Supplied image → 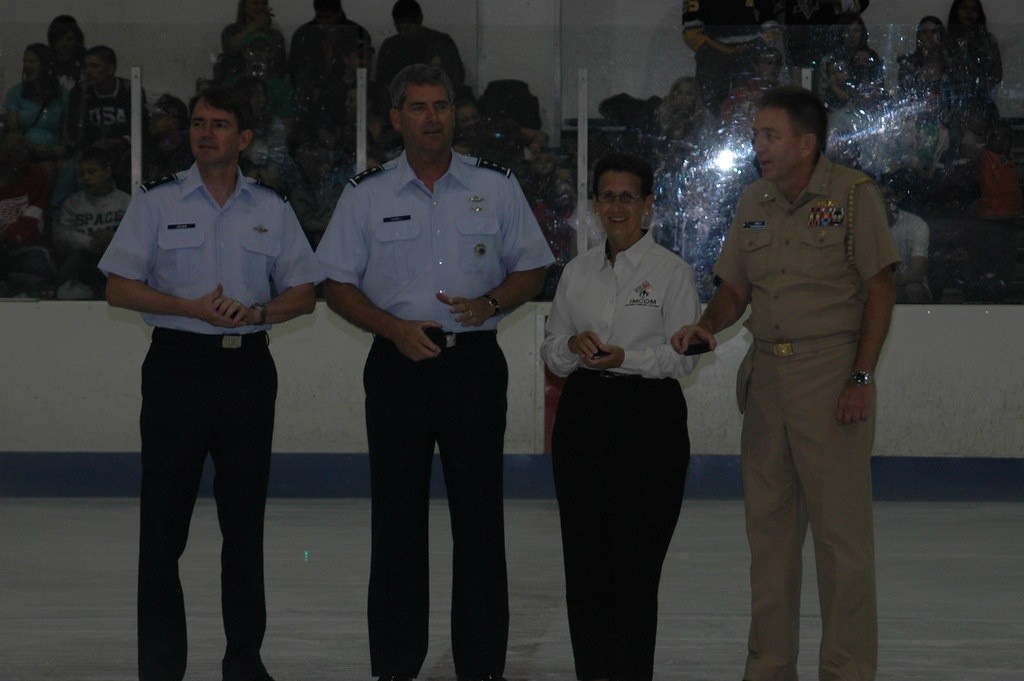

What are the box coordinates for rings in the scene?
[469,309,473,316]
[234,301,240,306]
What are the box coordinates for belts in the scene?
[754,330,857,359]
[577,366,641,379]
[374,327,498,348]
[151,325,266,349]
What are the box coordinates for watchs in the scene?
[850,371,872,386]
[483,294,499,316]
[252,302,265,325]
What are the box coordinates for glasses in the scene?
[598,190,641,204]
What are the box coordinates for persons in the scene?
[98,88,326,681]
[1,0,1024,305]
[310,62,555,681]
[670,86,903,681]
[540,154,703,681]
